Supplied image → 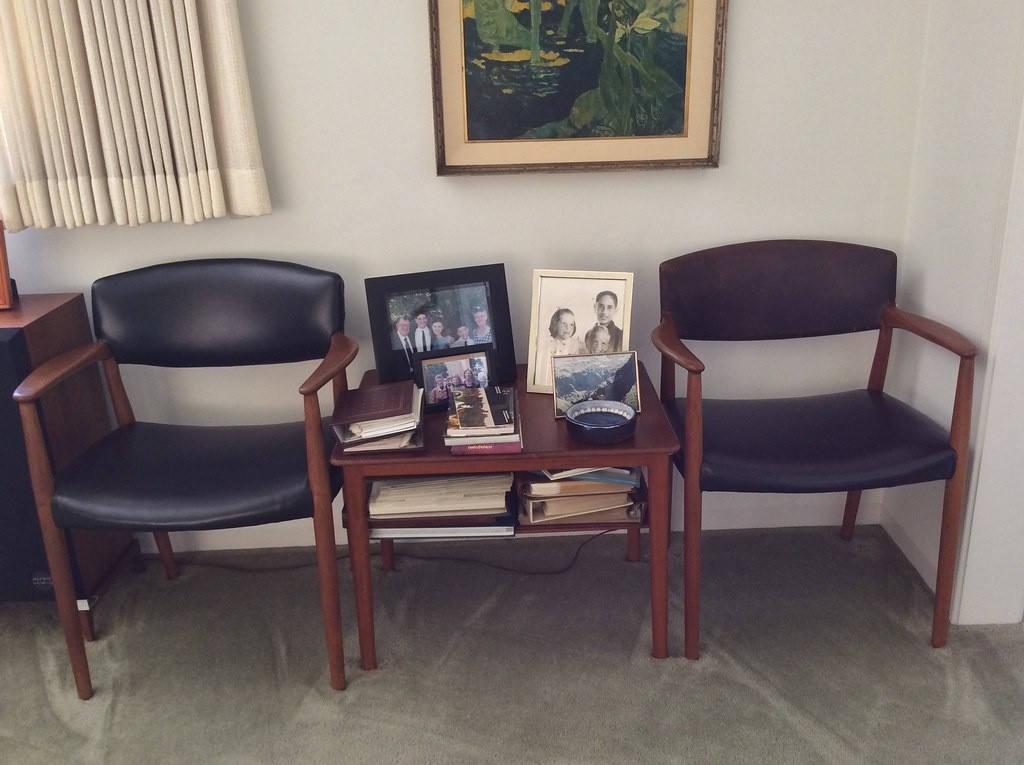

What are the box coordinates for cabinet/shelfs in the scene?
[0,292,147,644]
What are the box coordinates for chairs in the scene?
[14,256,359,701]
[649,239,978,659]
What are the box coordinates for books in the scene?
[443,387,524,454]
[329,382,424,453]
[366,467,643,538]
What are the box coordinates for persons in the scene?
[584,291,622,354]
[431,370,489,404]
[391,305,493,380]
[535,309,578,387]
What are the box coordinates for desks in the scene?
[328,361,682,667]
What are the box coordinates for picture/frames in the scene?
[364,262,518,386]
[526,269,635,394]
[552,350,642,419]
[428,0,730,178]
[410,343,498,414]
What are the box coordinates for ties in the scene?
[404,338,414,366]
[465,341,467,346]
[422,330,427,351]
